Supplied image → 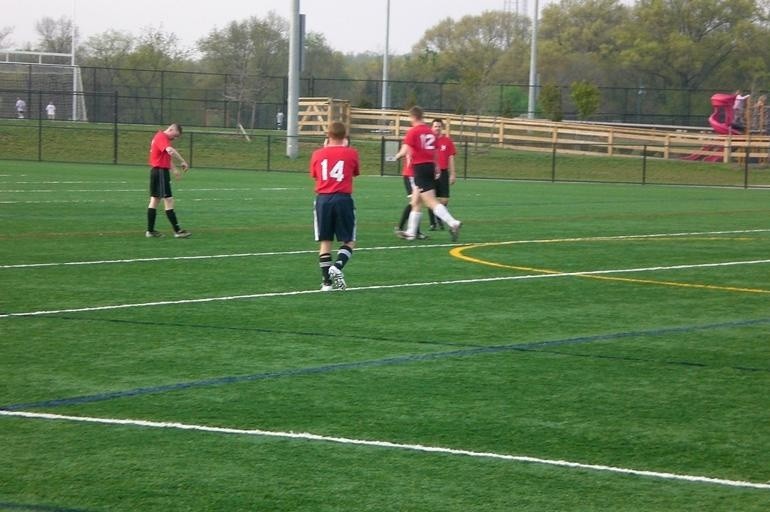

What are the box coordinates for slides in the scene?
[678,94,744,161]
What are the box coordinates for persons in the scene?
[385,137,431,239]
[311,123,360,291]
[276,109,285,130]
[145,123,192,237]
[397,106,462,241]
[45,102,56,119]
[427,119,456,231]
[15,97,25,118]
[733,89,750,122]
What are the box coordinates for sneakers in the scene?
[328,265,347,291]
[396,231,417,240]
[449,220,462,241]
[429,218,444,231]
[417,232,426,239]
[146,230,164,237]
[320,283,332,290]
[174,229,192,238]
